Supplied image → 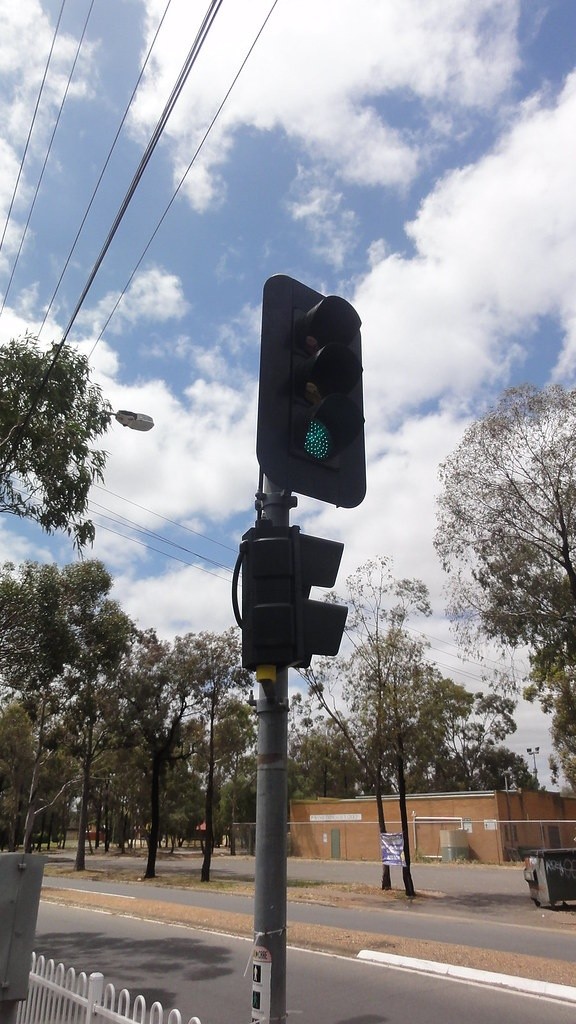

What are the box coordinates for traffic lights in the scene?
[232,519,350,672]
[256,274,366,509]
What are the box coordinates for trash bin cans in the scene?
[520,849,576,908]
